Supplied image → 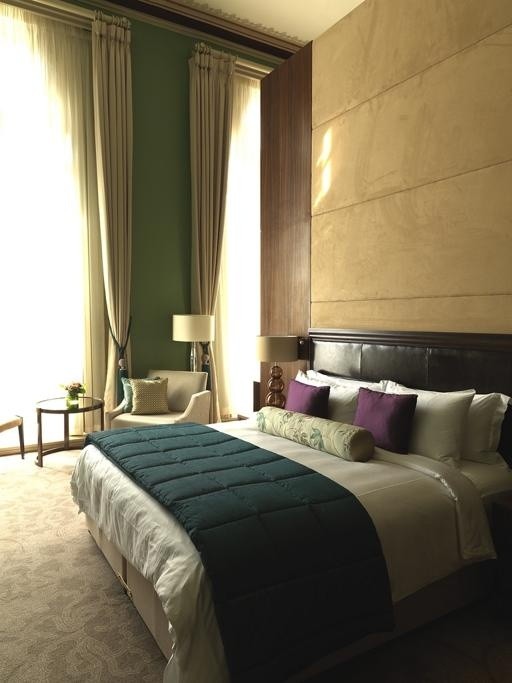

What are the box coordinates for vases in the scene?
[66,395,79,410]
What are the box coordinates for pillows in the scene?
[120,376,170,415]
[255,368,511,468]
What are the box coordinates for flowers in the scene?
[63,381,85,395]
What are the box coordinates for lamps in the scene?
[255,335,298,408]
[170,314,216,373]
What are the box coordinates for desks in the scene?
[35,395,105,468]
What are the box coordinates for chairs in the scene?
[0,413,27,459]
[103,368,211,433]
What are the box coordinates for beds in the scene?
[83,327,511,682]
